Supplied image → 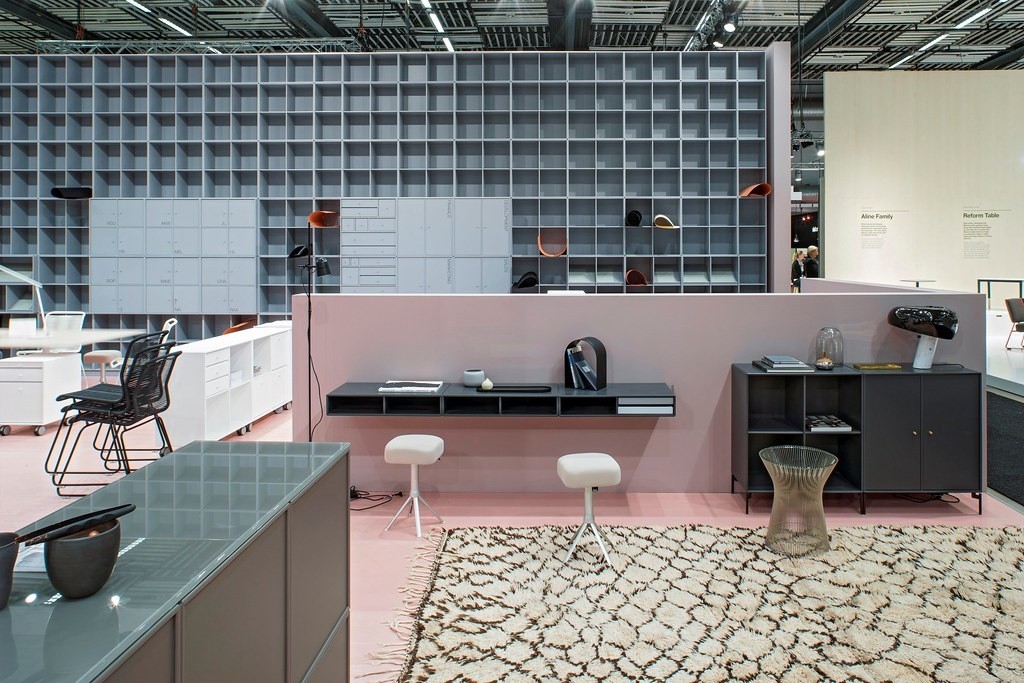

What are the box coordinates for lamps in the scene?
[712,5,739,48]
[794,215,799,243]
[297,222,332,442]
[791,130,825,181]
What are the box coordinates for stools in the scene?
[382,434,444,536]
[557,452,621,567]
[83,349,122,385]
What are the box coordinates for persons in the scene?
[792,251,805,293]
[805,246,819,277]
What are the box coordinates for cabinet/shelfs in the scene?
[731,361,982,516]
[0,438,352,683]
[0,50,767,380]
[0,352,82,437]
[156,318,293,456]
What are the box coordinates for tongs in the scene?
[14,503,137,547]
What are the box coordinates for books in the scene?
[807,415,852,432]
[379,381,443,392]
[752,356,815,372]
[567,346,596,390]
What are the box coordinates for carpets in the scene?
[355,524,1024,683]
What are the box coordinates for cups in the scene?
[0,532,19,610]
[43,519,120,598]
[463,369,485,387]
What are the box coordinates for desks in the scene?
[900,279,936,288]
[326,381,677,418]
[978,277,1024,310]
[0,327,146,349]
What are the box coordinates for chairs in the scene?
[14,310,89,390]
[1005,298,1024,350]
[45,329,184,496]
